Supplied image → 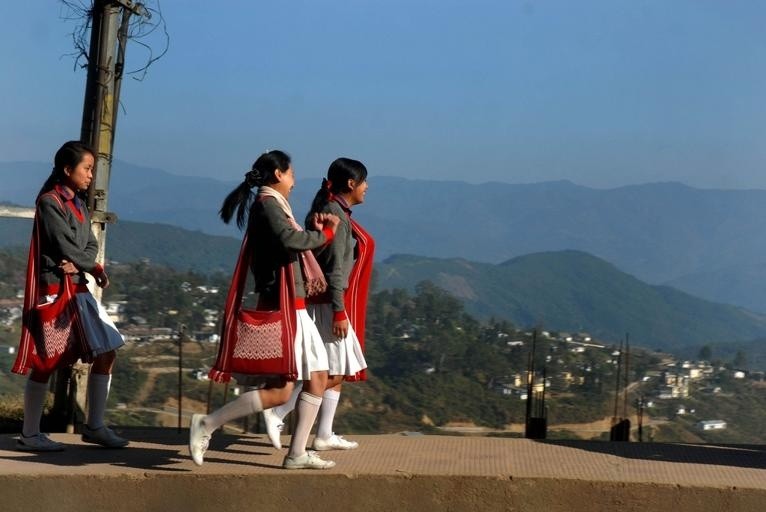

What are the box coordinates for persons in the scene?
[261,158,375,450]
[22,140,129,452]
[190,151,342,469]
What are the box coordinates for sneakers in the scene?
[262,408,359,469]
[189,414,210,466]
[16,432,65,451]
[82,424,129,448]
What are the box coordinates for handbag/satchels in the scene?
[10,291,93,375]
[208,309,298,384]
